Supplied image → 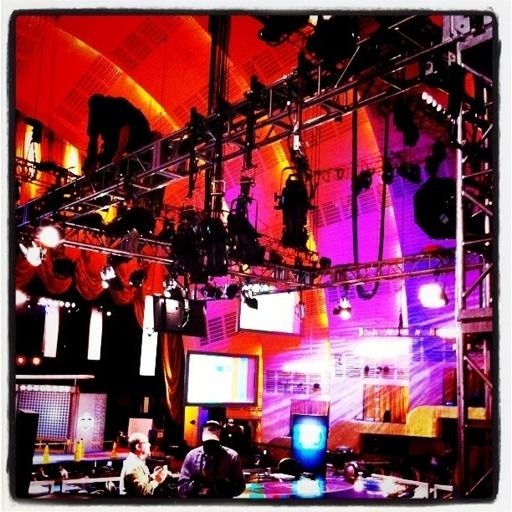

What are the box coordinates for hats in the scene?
[201,420,224,442]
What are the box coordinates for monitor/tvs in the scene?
[236,281,303,335]
[186,350,258,406]
[153,296,208,338]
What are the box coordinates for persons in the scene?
[178,419,248,503]
[118,433,168,503]
